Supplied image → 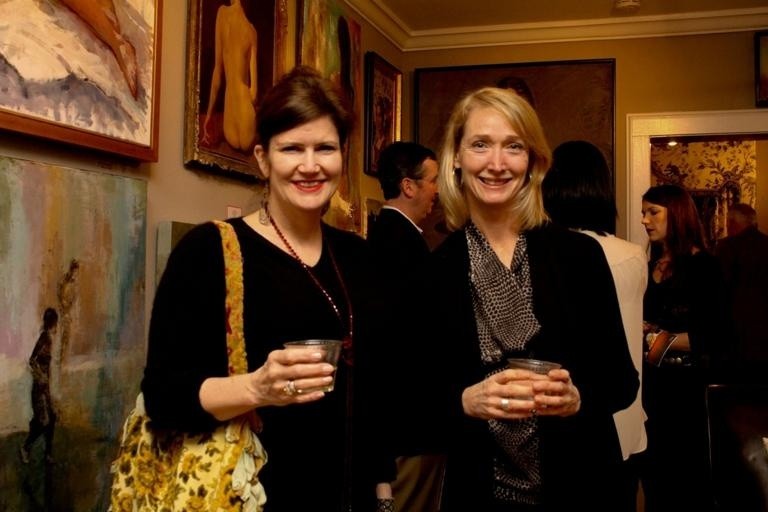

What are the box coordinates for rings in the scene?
[282,379,297,396]
[501,398,510,410]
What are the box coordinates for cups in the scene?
[508,358,563,414]
[284,339,343,393]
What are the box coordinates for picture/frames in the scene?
[0,0,164,163]
[361,51,618,187]
[183,2,289,185]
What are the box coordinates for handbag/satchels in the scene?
[106,219,268,512]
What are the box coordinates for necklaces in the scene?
[253,192,360,372]
[656,260,672,272]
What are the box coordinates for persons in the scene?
[134,63,411,512]
[402,83,646,512]
[364,139,459,509]
[201,1,264,158]
[634,182,718,511]
[705,201,767,407]
[55,0,140,102]
[536,137,652,510]
[19,306,66,474]
